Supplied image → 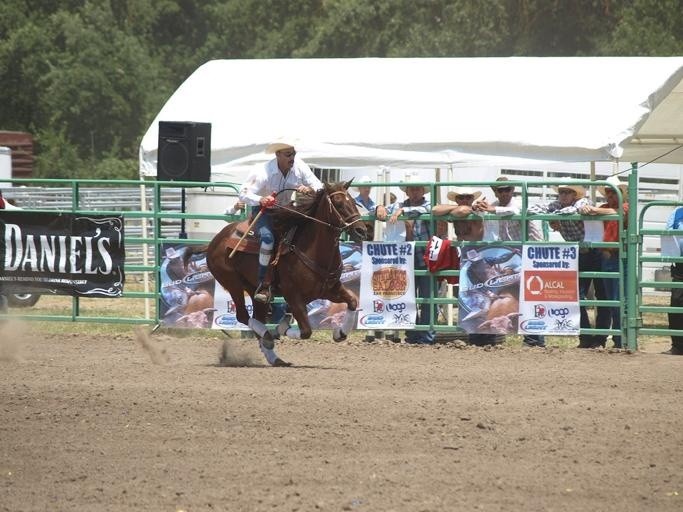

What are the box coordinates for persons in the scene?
[224,176,629,354]
[659,204,683,355]
[237,132,325,305]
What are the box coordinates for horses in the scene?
[182,177,368,367]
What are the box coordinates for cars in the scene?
[0,188,41,309]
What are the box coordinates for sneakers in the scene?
[661,346,683,355]
[254,289,274,305]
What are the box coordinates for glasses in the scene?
[280,151,295,157]
[559,189,572,194]
[605,189,618,195]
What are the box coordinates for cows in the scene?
[462,289,523,333]
[319,285,365,329]
[174,285,219,328]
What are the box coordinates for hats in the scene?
[447,177,515,201]
[552,176,586,201]
[596,177,629,199]
[352,176,378,192]
[397,173,432,194]
[266,136,304,153]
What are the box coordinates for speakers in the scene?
[157,121,211,182]
